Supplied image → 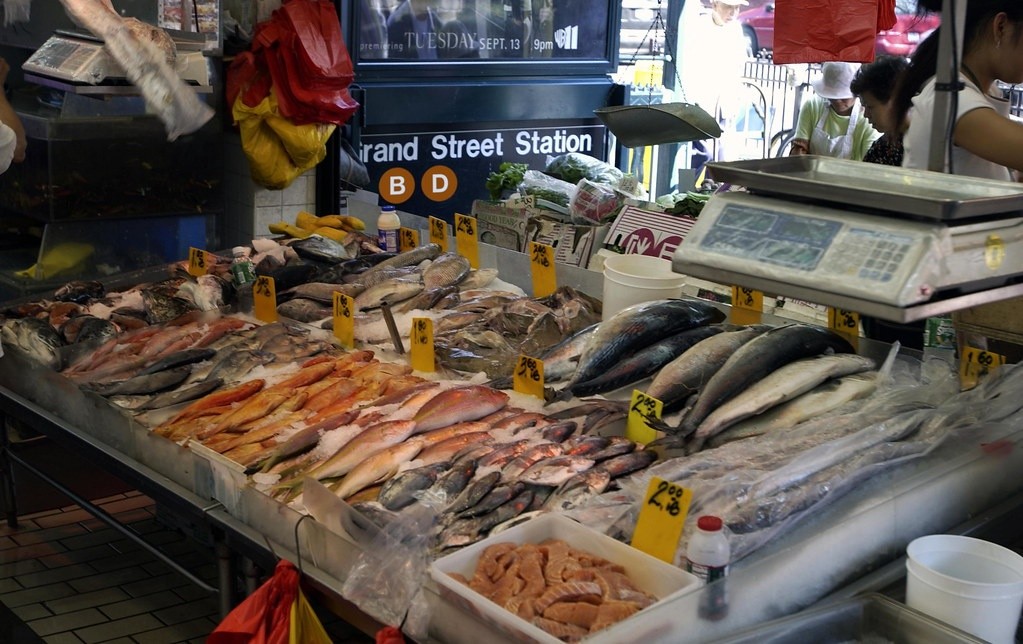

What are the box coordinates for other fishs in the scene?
[413,386,511,435]
[0,288,326,416]
[611,358,1023,567]
[263,418,417,491]
[151,349,426,461]
[235,234,500,342]
[539,296,877,456]
[330,404,658,547]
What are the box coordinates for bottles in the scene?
[378,205,400,252]
[921,315,955,384]
[230,246,258,292]
[688,516,728,620]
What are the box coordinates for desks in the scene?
[0,386,235,620]
[206,506,418,644]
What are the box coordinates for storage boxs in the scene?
[0,217,223,301]
[428,511,703,644]
[0,90,225,222]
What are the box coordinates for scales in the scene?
[22,28,213,96]
[592,0,722,149]
[671,154,1023,325]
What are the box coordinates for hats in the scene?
[710,0,749,6]
[811,62,856,99]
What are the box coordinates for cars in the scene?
[738,0,941,60]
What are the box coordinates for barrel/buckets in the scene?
[905,534,1023,644]
[600,254,689,321]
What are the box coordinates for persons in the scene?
[790,59,884,163]
[890,1,1023,181]
[850,54,927,349]
[657,0,749,199]
[0,56,29,174]
